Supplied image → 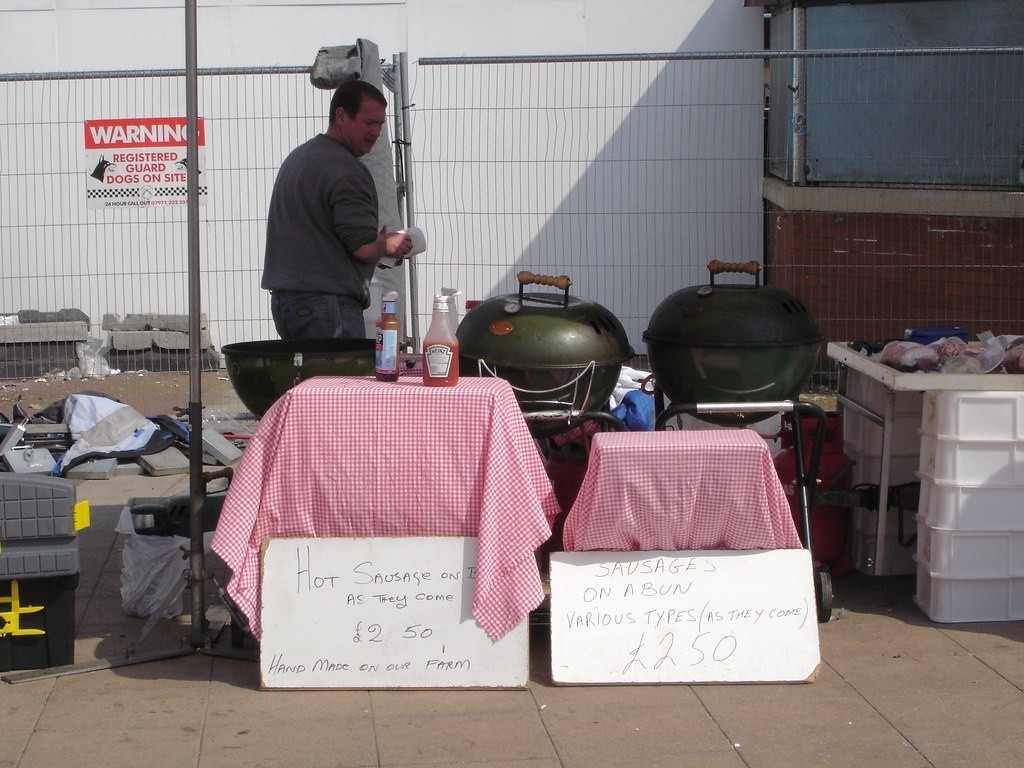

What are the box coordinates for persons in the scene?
[261,80,412,339]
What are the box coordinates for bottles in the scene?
[291,351,304,389]
[423,295,459,387]
[376,290,401,382]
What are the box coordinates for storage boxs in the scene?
[911,389,1024,624]
[826,341,1024,576]
[0,472,90,673]
[905,326,968,346]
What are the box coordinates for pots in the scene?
[637,260,826,429]
[455,271,635,438]
[222,338,407,421]
